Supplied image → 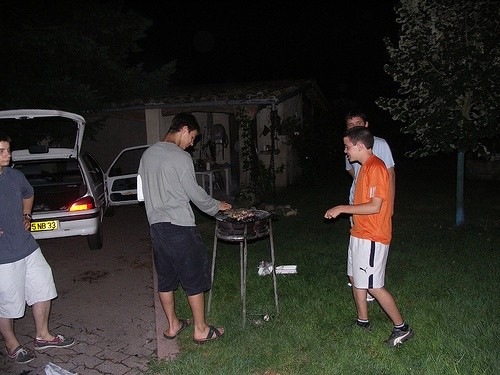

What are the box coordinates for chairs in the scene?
[56,161,79,173]
[16,163,42,178]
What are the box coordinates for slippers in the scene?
[163,318,190,339]
[193,325,222,344]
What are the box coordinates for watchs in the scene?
[24,214,32,219]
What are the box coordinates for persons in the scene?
[324,110,415,349]
[0,134,75,364]
[138,112,232,344]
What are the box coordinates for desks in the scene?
[195,166,230,198]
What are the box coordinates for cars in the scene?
[0,109,151,250]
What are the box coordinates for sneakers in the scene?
[354,318,371,329]
[383,323,414,347]
[4,344,37,364]
[35,333,75,350]
